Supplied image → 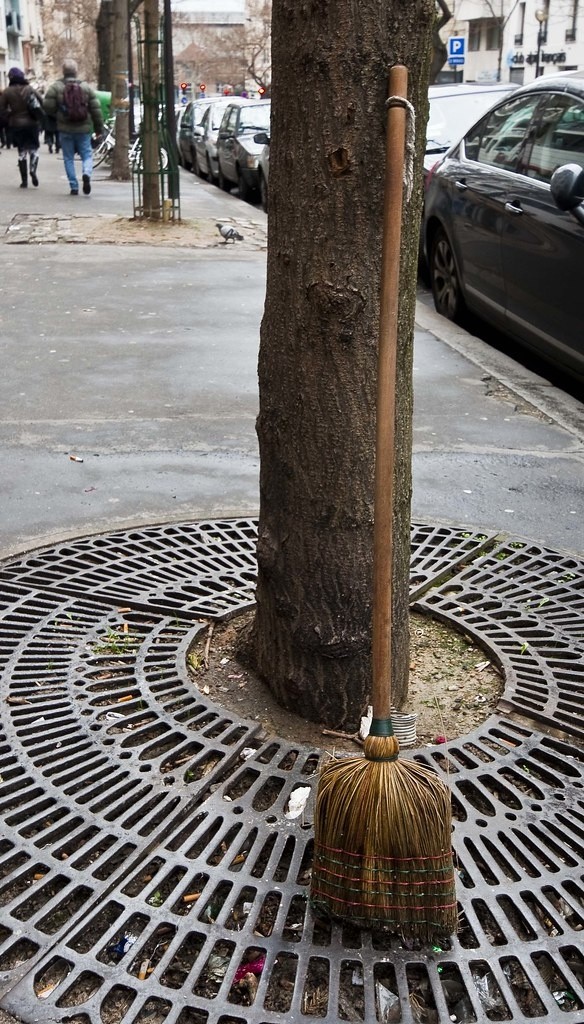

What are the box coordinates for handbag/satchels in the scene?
[26,86,44,121]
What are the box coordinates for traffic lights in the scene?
[258,88,266,95]
[200,85,206,90]
[181,83,186,89]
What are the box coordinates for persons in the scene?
[0,67,61,188]
[42,60,104,194]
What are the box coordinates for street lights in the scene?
[535,10,547,77]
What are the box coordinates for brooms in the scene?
[305,61,460,942]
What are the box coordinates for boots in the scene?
[29,153,39,186]
[18,159,28,188]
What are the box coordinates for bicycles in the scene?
[92,120,168,171]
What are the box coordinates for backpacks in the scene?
[57,78,88,121]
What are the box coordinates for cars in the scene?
[419,71,584,380]
[133,104,166,125]
[520,129,584,179]
[193,102,228,181]
[176,96,245,170]
[217,99,272,212]
[252,123,271,214]
[421,80,524,174]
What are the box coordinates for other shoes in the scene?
[48,143,60,153]
[82,174,91,194]
[0,142,11,149]
[71,189,78,195]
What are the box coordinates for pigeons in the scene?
[214,222,244,245]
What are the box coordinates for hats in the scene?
[62,59,78,75]
[8,67,24,79]
